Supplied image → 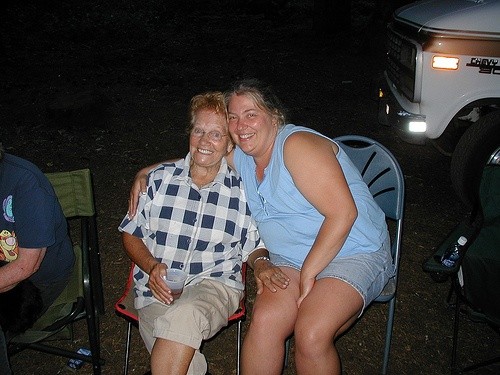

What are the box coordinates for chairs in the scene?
[116,262,246,375]
[421,163,500,375]
[6,169,106,375]
[282,135,404,374]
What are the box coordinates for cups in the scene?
[160,268,187,306]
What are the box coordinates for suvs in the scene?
[379,0,500,217]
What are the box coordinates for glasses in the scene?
[190,127,231,141]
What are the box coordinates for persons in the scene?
[118,94,290,375]
[0,144,75,341]
[128,80,393,375]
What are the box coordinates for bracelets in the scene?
[254,256,269,264]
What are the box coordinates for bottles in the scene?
[430,236,467,284]
